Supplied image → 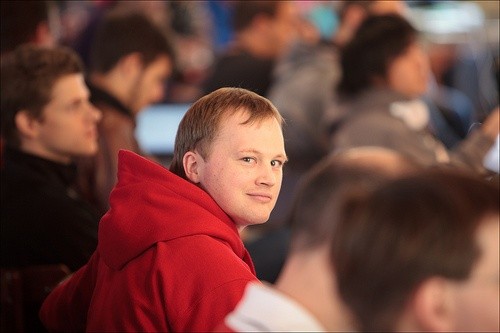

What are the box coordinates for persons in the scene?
[0,42,104,333]
[207,144,424,333]
[1,0,500,172]
[62,7,175,217]
[37,86,289,333]
[241,1,410,286]
[328,165,499,333]
